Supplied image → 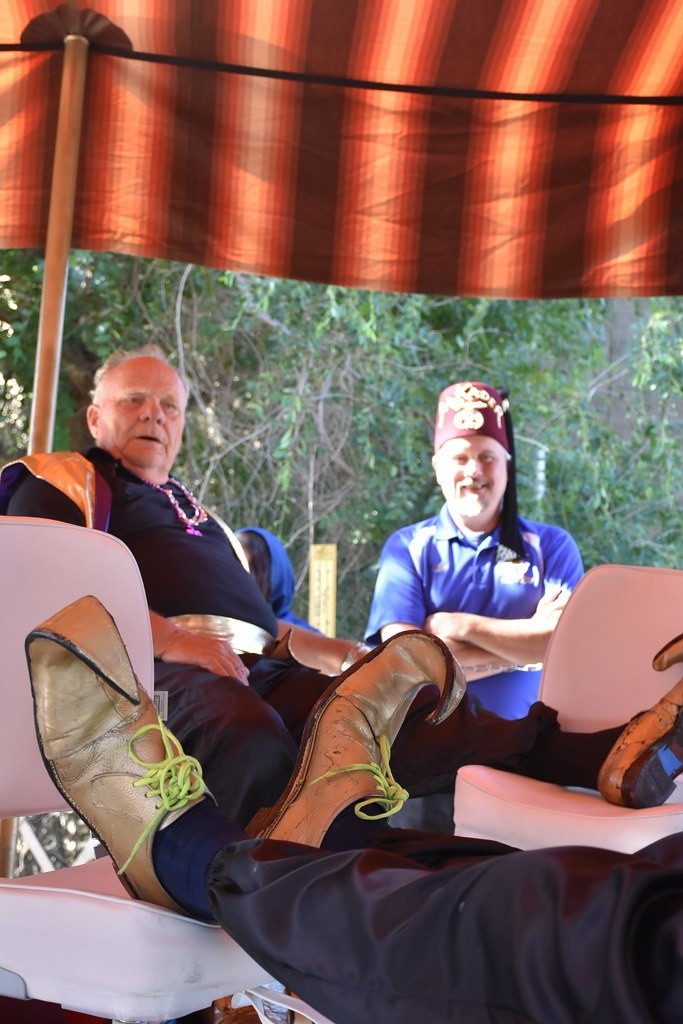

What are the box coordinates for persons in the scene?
[363,380,585,720]
[0,344,683,830]
[234,527,322,636]
[25,596,683,1024]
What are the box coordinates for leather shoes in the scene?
[596,632,683,809]
[238,629,468,848]
[25,596,224,922]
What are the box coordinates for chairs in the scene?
[452,564,683,854]
[0,513,290,1024]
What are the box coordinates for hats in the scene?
[434,382,510,453]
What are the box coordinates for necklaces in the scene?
[141,477,205,536]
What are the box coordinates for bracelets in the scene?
[341,641,364,671]
